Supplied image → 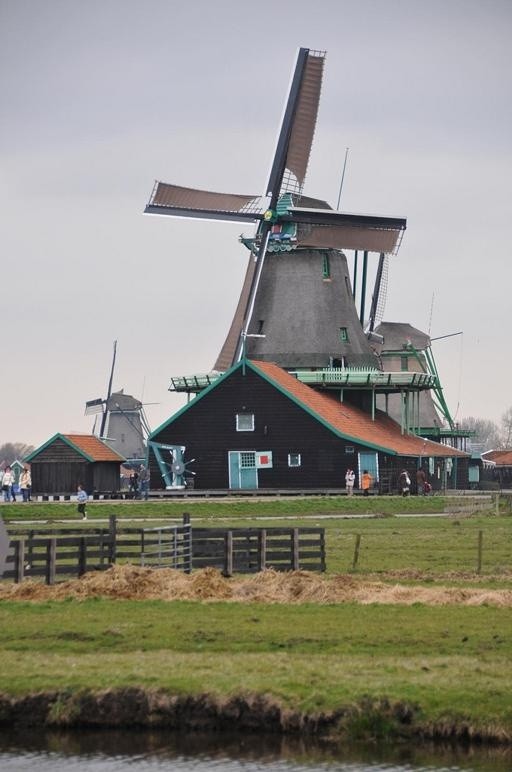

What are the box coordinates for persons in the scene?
[77,485,88,521]
[345,467,355,496]
[361,470,372,496]
[399,468,411,496]
[129,469,138,500]
[1,468,15,503]
[416,467,426,496]
[19,466,33,502]
[136,464,151,500]
[7,465,16,502]
